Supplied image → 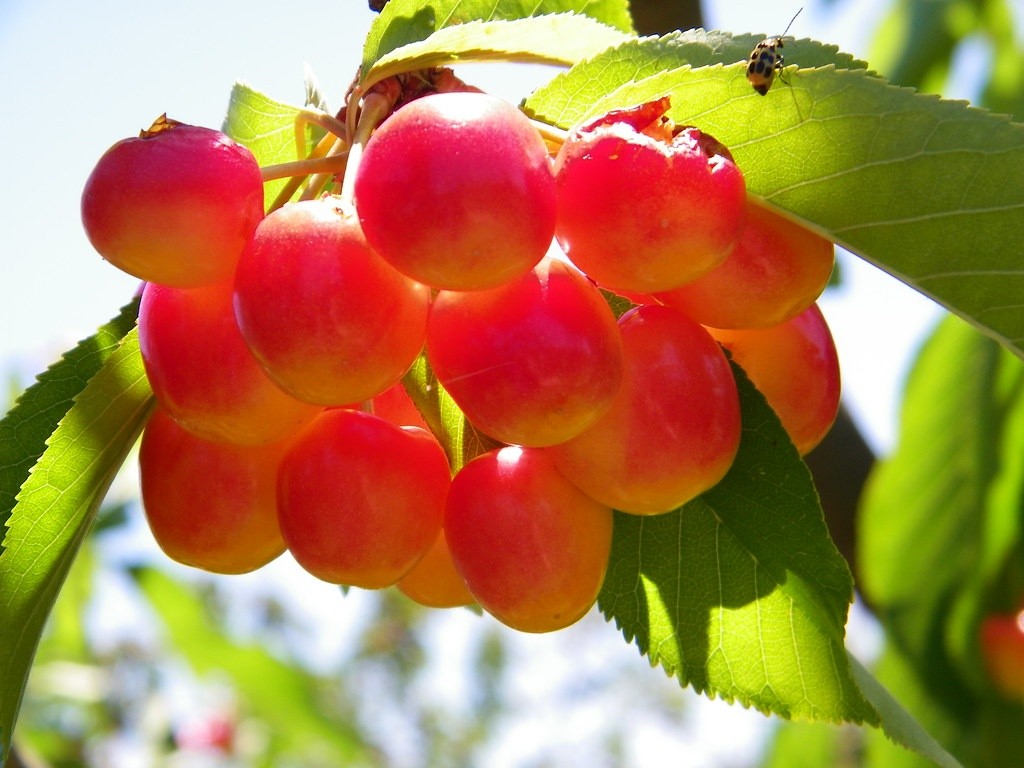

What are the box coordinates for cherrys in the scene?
[79,66,844,634]
